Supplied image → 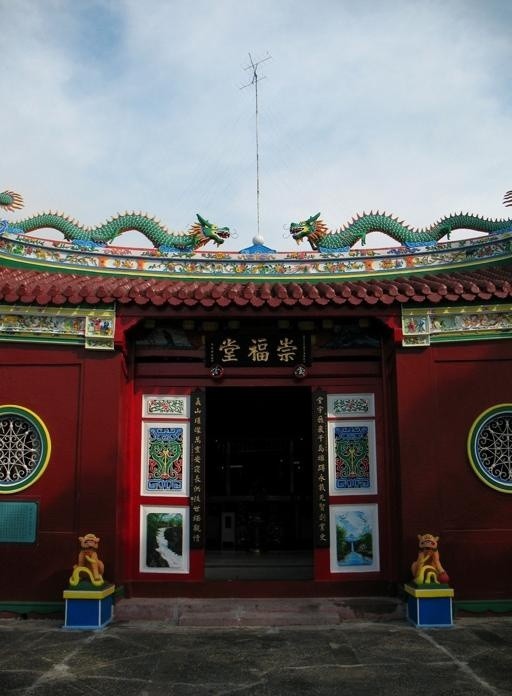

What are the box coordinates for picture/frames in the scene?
[328,503,381,574]
[141,394,192,419]
[326,392,376,419]
[139,504,190,575]
[140,421,191,498]
[327,420,378,497]
